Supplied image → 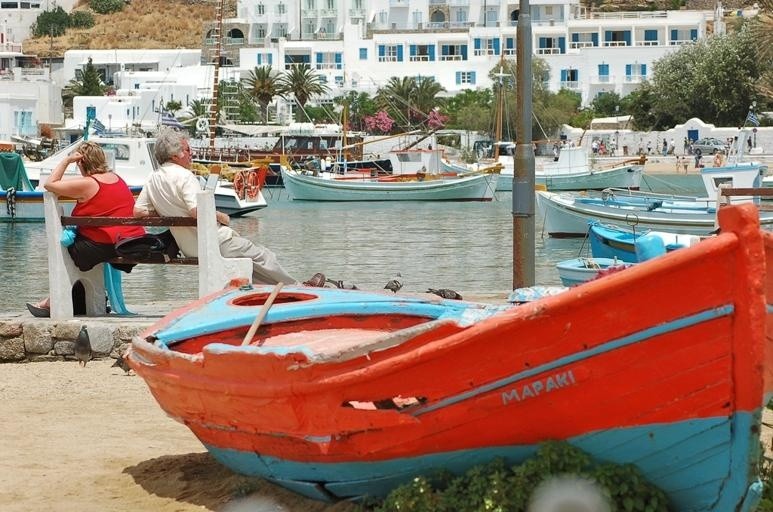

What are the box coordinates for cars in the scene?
[692,138,729,155]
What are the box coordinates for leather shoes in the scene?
[303,272,326,290]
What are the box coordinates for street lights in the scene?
[749,101,757,148]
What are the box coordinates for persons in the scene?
[25,139,147,318]
[591,135,753,175]
[132,130,326,288]
[553,138,575,162]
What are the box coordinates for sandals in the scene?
[26,301,54,317]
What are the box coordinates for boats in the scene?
[0,134,267,223]
[247,158,392,188]
[535,163,773,287]
[123,202,773,512]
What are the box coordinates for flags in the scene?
[746,111,761,127]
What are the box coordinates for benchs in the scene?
[40,190,254,319]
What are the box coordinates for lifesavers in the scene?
[246,172,261,199]
[233,172,245,199]
[714,154,723,168]
[195,118,209,132]
[278,103,287,115]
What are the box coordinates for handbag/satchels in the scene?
[115,228,181,260]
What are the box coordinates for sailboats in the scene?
[135,0,363,167]
[279,42,505,201]
[439,38,649,193]
